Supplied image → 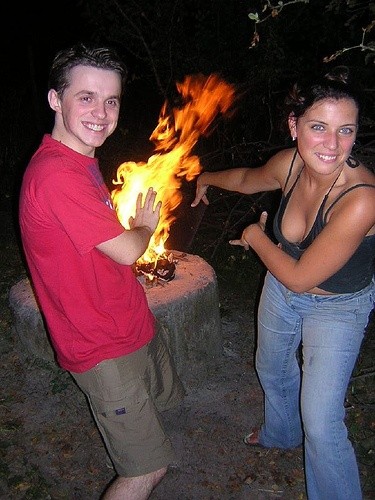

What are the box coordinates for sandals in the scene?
[243,429,261,446]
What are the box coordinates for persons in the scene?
[18,44,187,500]
[190,84,375,500]
